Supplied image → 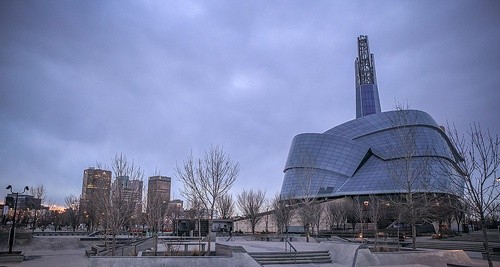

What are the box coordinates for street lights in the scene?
[176,202,181,236]
[0,185,29,254]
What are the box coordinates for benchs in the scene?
[387,242,412,246]
[167,242,206,256]
[262,236,293,242]
